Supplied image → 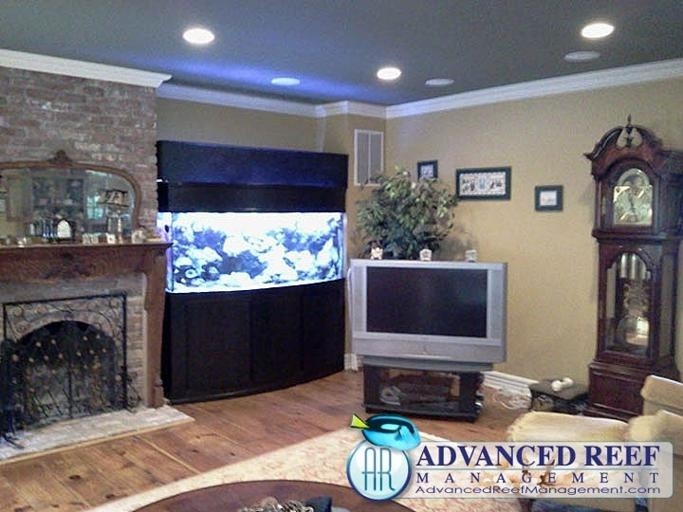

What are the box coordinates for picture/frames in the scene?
[534,185,562,211]
[455,166,511,200]
[416,160,437,183]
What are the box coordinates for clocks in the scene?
[582,114,682,423]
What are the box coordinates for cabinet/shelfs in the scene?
[160,277,347,405]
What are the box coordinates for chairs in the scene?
[509,374,683,511]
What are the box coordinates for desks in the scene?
[356,356,492,425]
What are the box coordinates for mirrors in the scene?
[0,150,142,245]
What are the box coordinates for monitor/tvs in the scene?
[348,259,509,363]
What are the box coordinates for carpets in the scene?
[81,425,521,511]
